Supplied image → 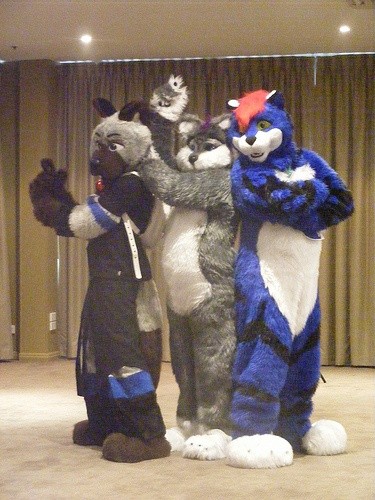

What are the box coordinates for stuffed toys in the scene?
[140,113,240,462]
[29,75,190,463]
[228,91,354,470]
